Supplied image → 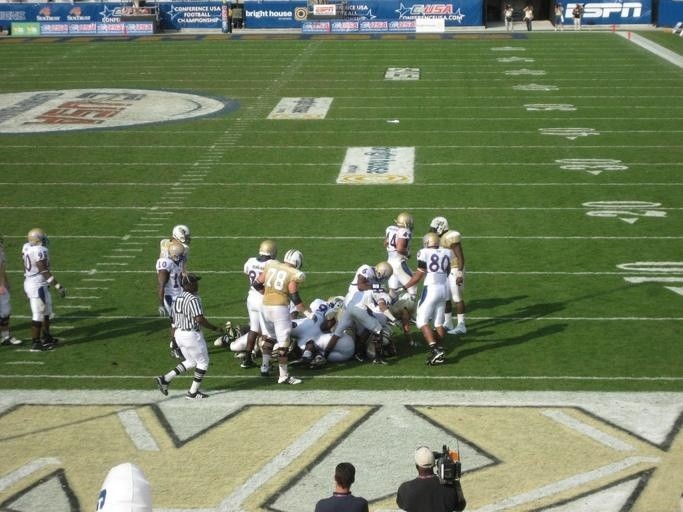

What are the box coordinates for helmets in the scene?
[395,212,414,227]
[168,243,184,262]
[283,249,302,268]
[172,224,190,243]
[375,262,392,281]
[258,240,276,257]
[431,216,448,235]
[28,228,47,246]
[423,232,440,249]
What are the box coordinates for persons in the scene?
[153,271,224,400]
[403,233,452,365]
[159,225,192,271]
[572,4,584,31]
[93,461,154,512]
[0,237,23,346]
[430,217,467,334]
[307,262,393,367]
[504,2,514,31]
[260,249,303,385]
[215,288,416,365]
[21,228,65,352]
[313,462,368,512]
[554,2,563,31]
[383,212,418,304]
[155,241,185,361]
[241,241,279,368]
[522,4,534,31]
[395,446,466,512]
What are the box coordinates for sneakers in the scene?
[425,348,444,365]
[373,356,388,364]
[1,337,23,345]
[214,320,326,385]
[433,322,455,330]
[447,326,467,335]
[155,374,168,396]
[31,336,58,350]
[185,389,208,399]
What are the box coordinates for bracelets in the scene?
[54,283,62,289]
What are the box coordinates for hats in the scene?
[180,273,201,286]
[415,447,435,469]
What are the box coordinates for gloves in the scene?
[54,284,65,298]
[158,306,165,317]
